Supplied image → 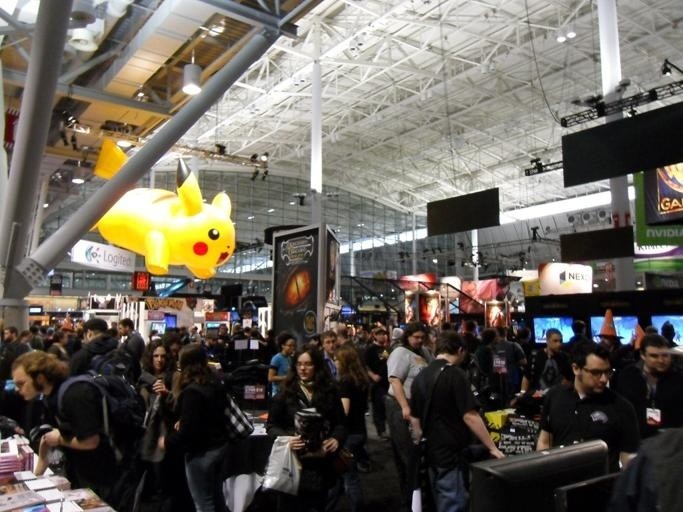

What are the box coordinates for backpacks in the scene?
[55,369,145,449]
[88,349,133,379]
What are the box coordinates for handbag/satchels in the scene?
[408,362,454,463]
[224,396,254,444]
[331,447,352,474]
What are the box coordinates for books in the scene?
[0,435,80,509]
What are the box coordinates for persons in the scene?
[176,324,258,357]
[409,331,504,512]
[158,342,229,493]
[136,341,180,475]
[71,318,140,384]
[567,310,645,357]
[383,324,434,502]
[621,334,682,436]
[10,350,148,511]
[116,318,144,354]
[0,318,79,363]
[268,347,346,492]
[317,331,340,386]
[147,329,161,339]
[334,347,368,512]
[330,316,383,342]
[365,328,398,440]
[482,329,531,408]
[536,342,640,457]
[516,328,573,396]
[267,331,299,396]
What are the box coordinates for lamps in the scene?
[556,26,576,43]
[662,59,683,78]
[72,160,86,184]
[182,51,203,96]
[63,110,77,123]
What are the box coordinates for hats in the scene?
[592,310,622,338]
[480,328,496,346]
[374,327,390,335]
[634,324,647,349]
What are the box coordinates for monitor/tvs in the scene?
[559,226,634,262]
[650,315,683,346]
[469,440,609,512]
[426,187,499,236]
[164,315,176,331]
[561,102,682,188]
[150,322,166,335]
[531,316,575,345]
[589,315,639,346]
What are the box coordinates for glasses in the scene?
[579,366,615,376]
